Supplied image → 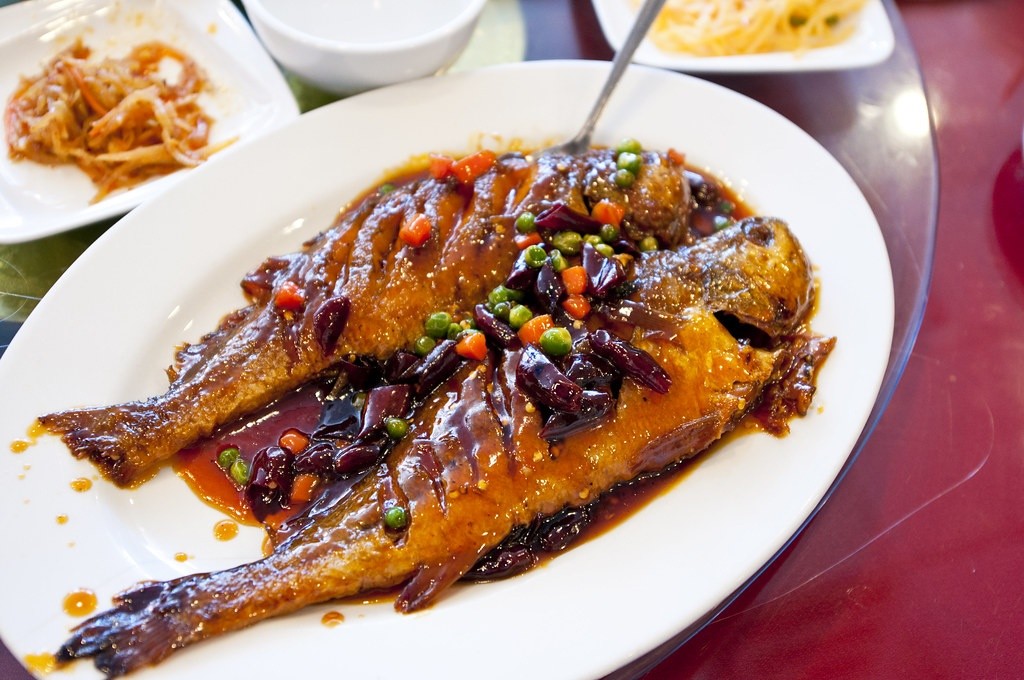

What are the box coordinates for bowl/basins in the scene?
[240,0,486,96]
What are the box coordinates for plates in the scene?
[0,58,897,680]
[0,0,303,246]
[591,0,896,74]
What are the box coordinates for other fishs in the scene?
[37,148,812,680]
